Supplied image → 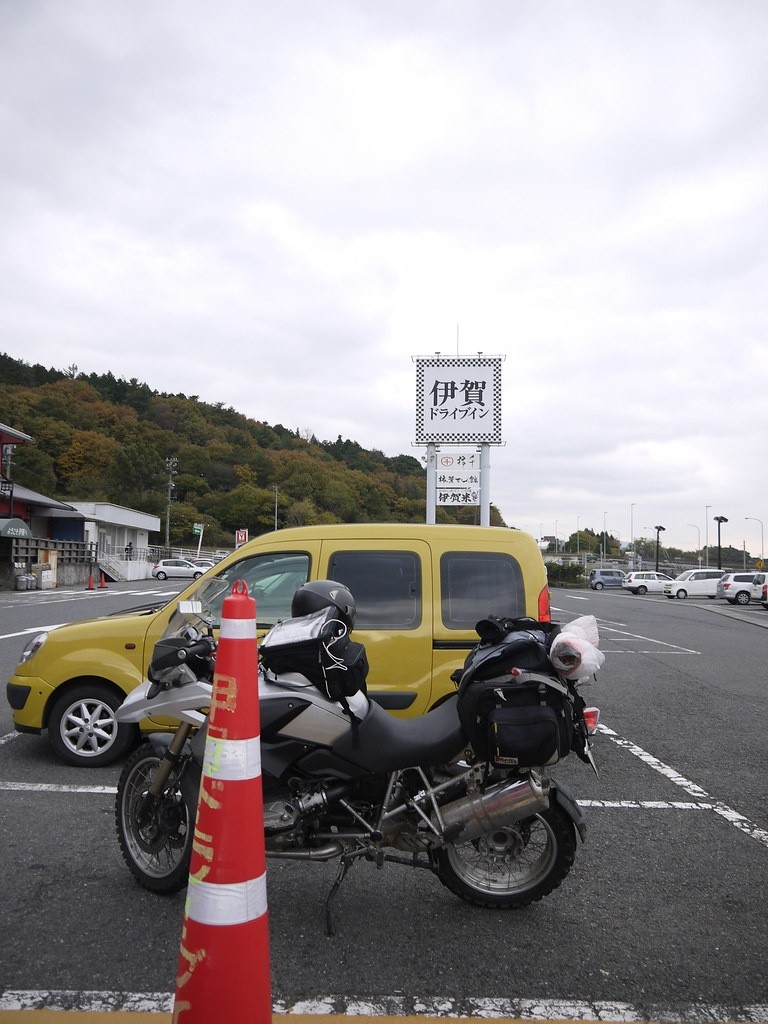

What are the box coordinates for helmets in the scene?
[292,579,357,633]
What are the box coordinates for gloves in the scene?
[501,635,568,686]
[475,614,516,643]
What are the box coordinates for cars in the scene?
[750,573,768,609]
[7,523,551,767]
[152,559,208,580]
[662,569,726,599]
[716,573,756,603]
[622,572,674,595]
[589,569,626,590]
[194,561,215,570]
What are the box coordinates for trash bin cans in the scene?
[15,573,36,591]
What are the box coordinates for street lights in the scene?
[705,506,713,568]
[655,526,665,572]
[745,518,764,563]
[687,524,701,569]
[631,503,636,571]
[165,471,177,548]
[714,516,728,570]
[555,512,608,564]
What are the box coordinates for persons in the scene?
[125,542,133,560]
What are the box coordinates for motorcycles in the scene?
[112,604,600,938]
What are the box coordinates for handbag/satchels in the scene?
[450,615,593,794]
[259,605,373,748]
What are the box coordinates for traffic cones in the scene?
[98,573,109,588]
[85,573,97,590]
[171,582,273,1024]
